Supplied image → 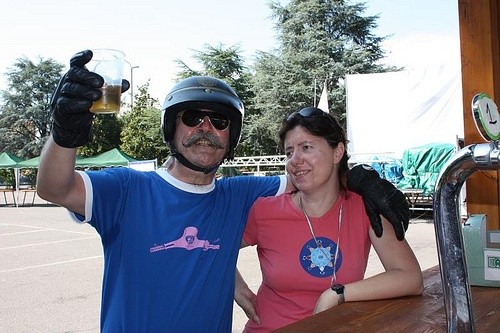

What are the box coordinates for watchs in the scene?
[331,283,345,306]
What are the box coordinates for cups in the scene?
[84,48,125,113]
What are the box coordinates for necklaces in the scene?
[299,192,342,287]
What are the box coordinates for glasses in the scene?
[282,107,332,124]
[175,110,238,132]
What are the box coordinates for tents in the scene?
[0,147,160,207]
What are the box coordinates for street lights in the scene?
[131,66,140,109]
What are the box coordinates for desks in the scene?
[21,189,38,206]
[0,188,17,207]
[268,266,500,333]
[399,189,424,218]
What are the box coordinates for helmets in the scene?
[161,76,245,151]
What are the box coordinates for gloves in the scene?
[344,163,410,242]
[51,49,130,148]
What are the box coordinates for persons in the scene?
[35,50,410,333]
[234,107,424,333]
